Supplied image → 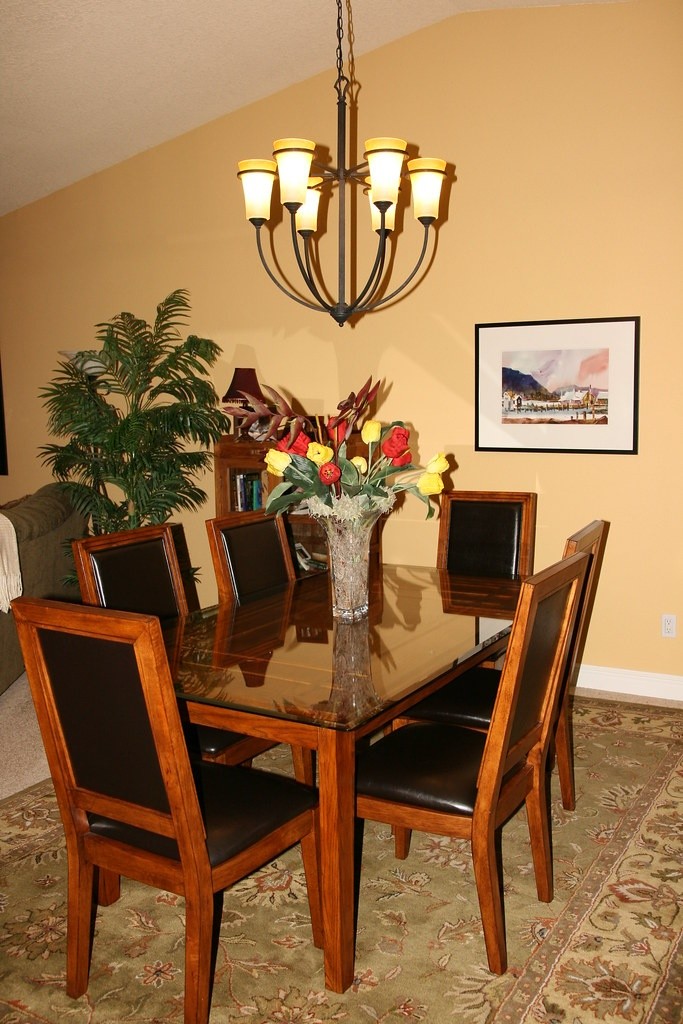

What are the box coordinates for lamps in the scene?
[220,367,265,442]
[234,0,448,329]
[235,651,274,687]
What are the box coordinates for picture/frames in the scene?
[475,316,641,455]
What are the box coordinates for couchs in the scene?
[0,480,100,695]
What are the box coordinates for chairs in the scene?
[11,595,325,1022]
[354,548,589,976]
[70,523,314,789]
[438,569,532,621]
[436,491,539,671]
[390,520,605,860]
[208,579,296,671]
[203,507,297,604]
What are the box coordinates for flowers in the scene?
[221,373,450,522]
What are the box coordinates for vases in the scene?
[312,616,384,717]
[311,506,384,620]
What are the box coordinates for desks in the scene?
[161,563,531,994]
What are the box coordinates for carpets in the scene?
[0,683,681,1023]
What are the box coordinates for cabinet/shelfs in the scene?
[214,428,383,575]
